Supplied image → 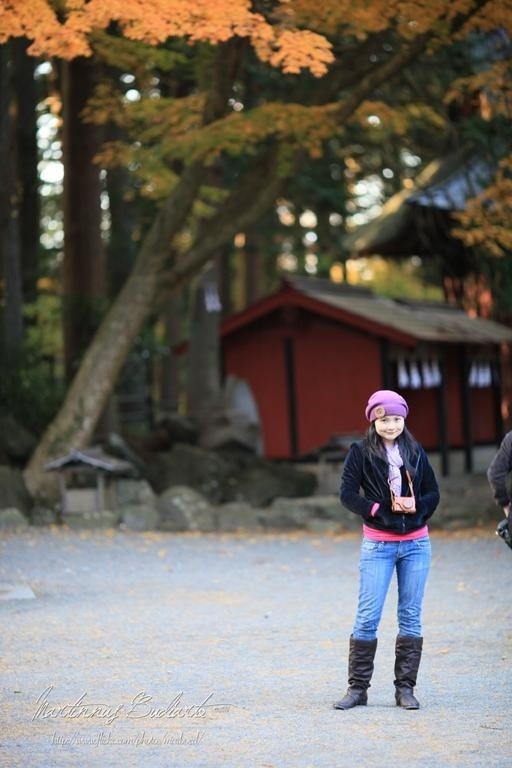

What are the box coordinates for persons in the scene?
[333,385,440,712]
[485,428,511,534]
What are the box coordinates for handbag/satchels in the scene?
[496,519,512,551]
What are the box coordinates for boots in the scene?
[394,633,423,709]
[335,635,378,709]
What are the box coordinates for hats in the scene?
[365,390,409,423]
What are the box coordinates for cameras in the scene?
[393,496,416,513]
